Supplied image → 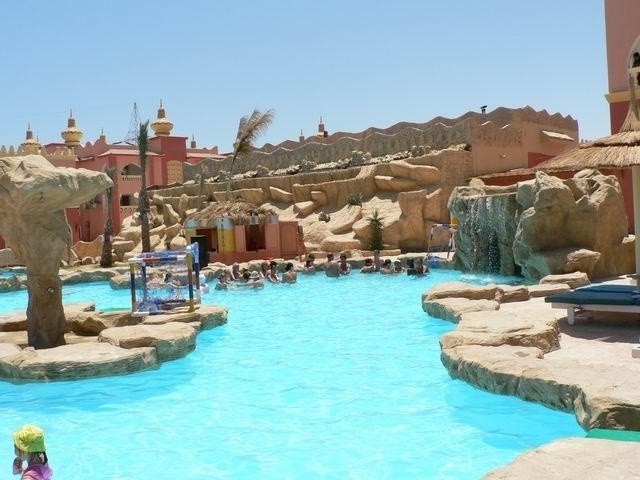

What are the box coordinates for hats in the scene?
[13,424,45,454]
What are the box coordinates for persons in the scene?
[7,423,54,480]
[157,248,430,294]
